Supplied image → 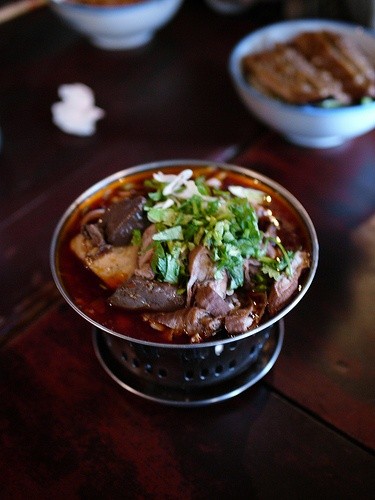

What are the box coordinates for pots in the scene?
[49,159,320,405]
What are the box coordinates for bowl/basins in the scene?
[229,19,375,148]
[49,0,182,52]
